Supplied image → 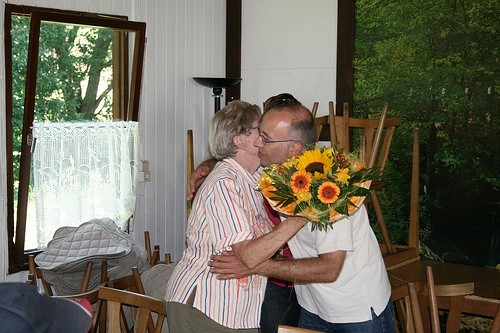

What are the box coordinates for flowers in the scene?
[257,146,392,232]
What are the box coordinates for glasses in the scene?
[259,133,295,145]
[262,93,299,108]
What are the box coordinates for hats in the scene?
[0,282,93,333]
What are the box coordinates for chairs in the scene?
[183,99,500,333]
[23,229,168,333]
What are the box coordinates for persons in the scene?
[162,100,310,333]
[190,98,397,333]
[258,92,301,333]
